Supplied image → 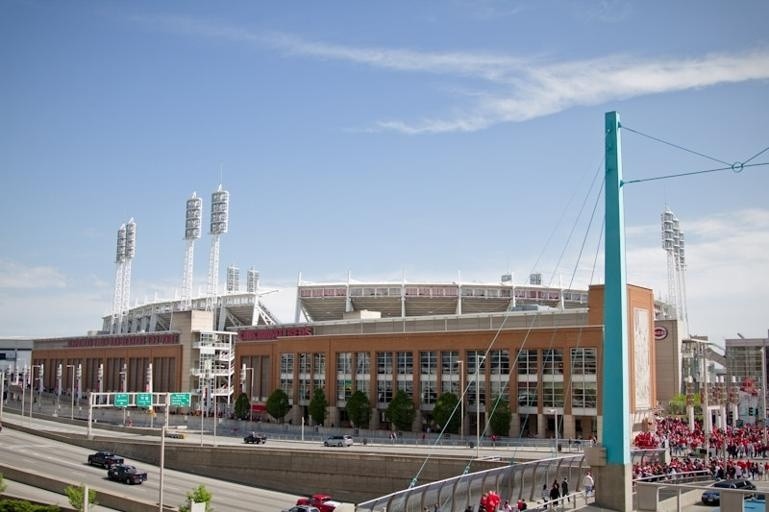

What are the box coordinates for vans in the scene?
[323,435,354,447]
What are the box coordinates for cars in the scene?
[282,505,320,512]
[244,434,267,445]
[702,478,757,506]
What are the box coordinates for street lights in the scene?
[198,364,225,448]
[455,359,464,442]
[246,368,254,431]
[158,423,188,512]
[0,364,75,434]
[118,372,127,425]
[476,355,487,457]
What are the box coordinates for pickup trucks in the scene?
[87,452,148,486]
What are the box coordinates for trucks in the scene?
[297,495,356,512]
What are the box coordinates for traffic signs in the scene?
[114,394,129,407]
[171,394,189,407]
[136,394,152,408]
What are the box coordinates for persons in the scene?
[461,475,572,512]
[583,471,595,497]
[630,412,769,481]
